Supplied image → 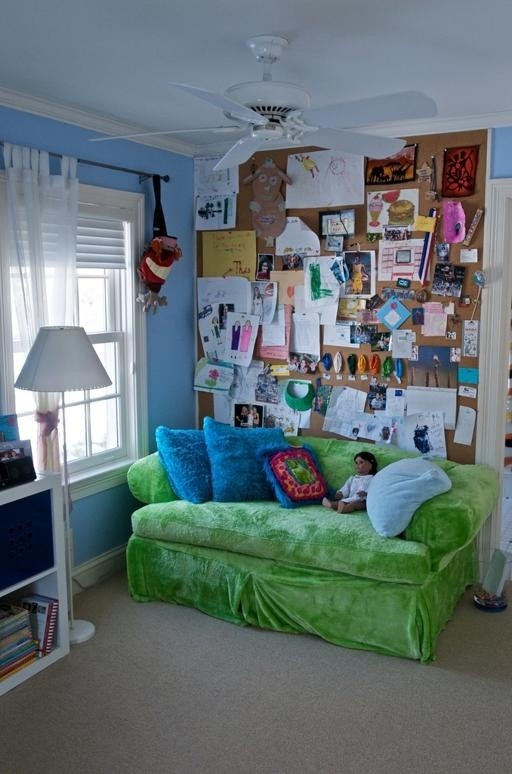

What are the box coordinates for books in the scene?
[0,593,59,683]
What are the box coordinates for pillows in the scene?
[255,442,331,508]
[155,425,213,503]
[202,415,288,502]
[364,455,453,538]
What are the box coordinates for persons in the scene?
[376,334,390,351]
[0,451,19,462]
[349,256,367,294]
[370,392,383,410]
[257,262,270,279]
[204,302,253,352]
[282,255,303,270]
[253,287,263,321]
[289,356,318,375]
[236,406,260,428]
[322,452,377,513]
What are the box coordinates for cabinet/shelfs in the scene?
[1,468,72,695]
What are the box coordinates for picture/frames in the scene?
[0,438,34,463]
[315,208,357,239]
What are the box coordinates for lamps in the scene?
[12,324,115,645]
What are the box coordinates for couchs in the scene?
[126,431,499,662]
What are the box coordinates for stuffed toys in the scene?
[136,235,183,314]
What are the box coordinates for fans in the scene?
[88,32,440,186]
